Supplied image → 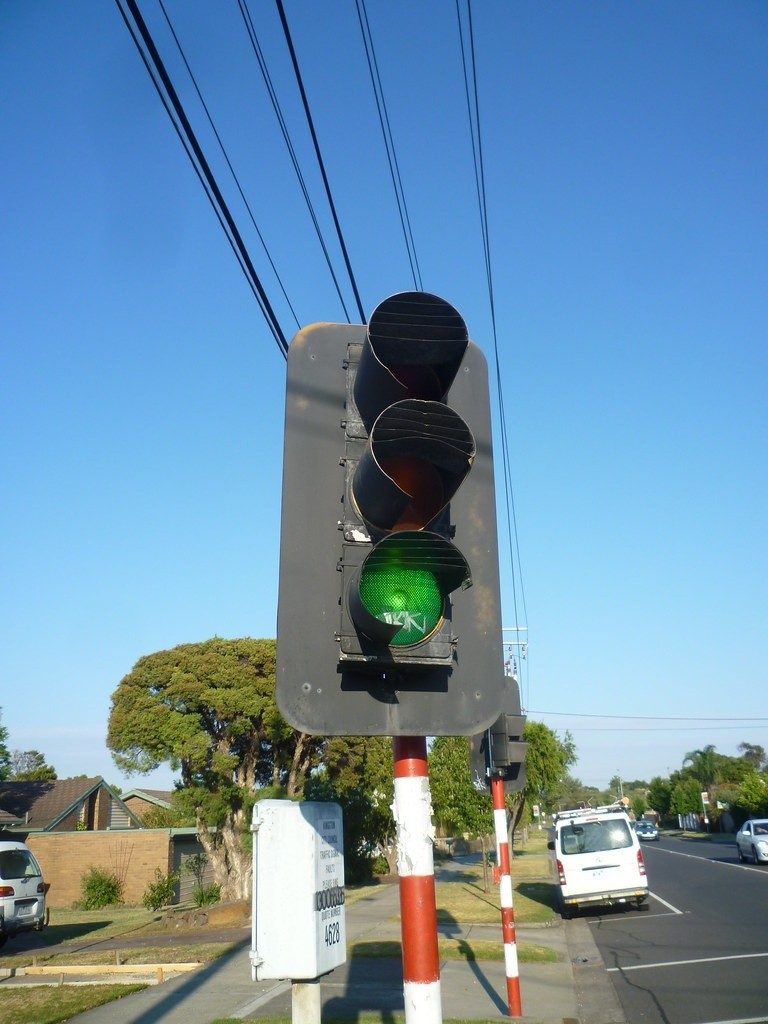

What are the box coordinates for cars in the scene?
[634,819,659,842]
[735,819,768,865]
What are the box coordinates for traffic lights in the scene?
[277,292,515,741]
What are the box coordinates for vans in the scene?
[547,800,651,918]
[0,841,48,943]
